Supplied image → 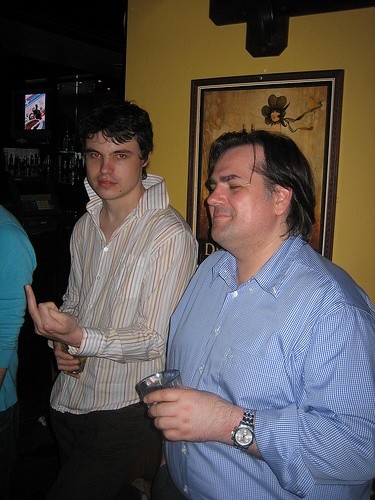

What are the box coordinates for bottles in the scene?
[9,153,40,166]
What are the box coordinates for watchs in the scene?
[233,406,252,457]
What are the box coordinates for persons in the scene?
[32,105,43,128]
[0,204,31,500]
[143,129,375,499]
[38,99,198,500]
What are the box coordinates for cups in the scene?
[135,370,183,408]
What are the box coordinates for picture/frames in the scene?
[186,70,345,267]
